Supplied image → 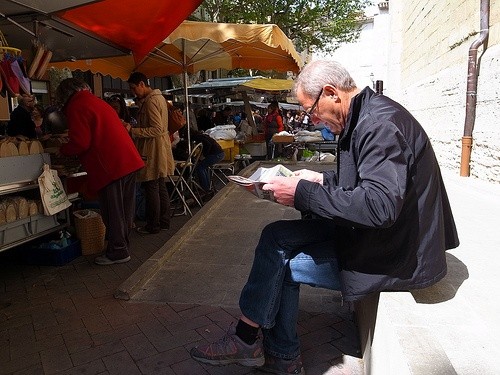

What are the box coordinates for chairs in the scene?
[209,160,235,193]
[165,142,203,218]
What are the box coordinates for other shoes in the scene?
[138,225,169,233]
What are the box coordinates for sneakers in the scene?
[191,328,265,366]
[254,352,305,375]
[94,250,130,265]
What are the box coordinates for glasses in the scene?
[107,96,120,103]
[303,84,337,118]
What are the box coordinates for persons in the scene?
[53,77,147,264]
[122,71,175,235]
[284,108,320,130]
[165,98,287,196]
[190,61,460,374]
[5,92,69,139]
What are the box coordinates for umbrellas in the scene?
[0,0,204,83]
[134,19,305,193]
